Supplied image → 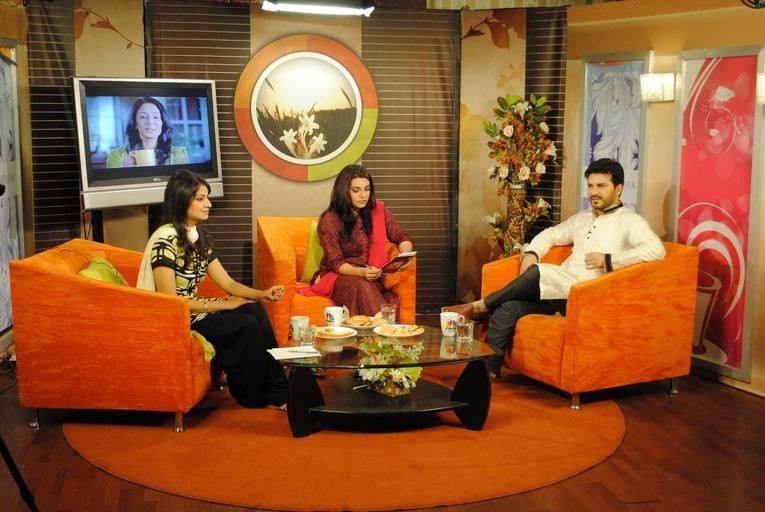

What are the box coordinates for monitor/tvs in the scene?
[70,73,225,212]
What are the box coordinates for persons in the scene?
[440,158,667,383]
[105,96,189,170]
[295,163,417,324]
[136,171,289,411]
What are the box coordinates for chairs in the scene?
[9,239,229,432]
[257,216,416,346]
[480,242,700,411]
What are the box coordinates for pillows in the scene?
[76,255,131,288]
[299,221,324,283]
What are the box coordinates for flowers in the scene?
[487,95,558,256]
[358,337,424,389]
[280,113,327,159]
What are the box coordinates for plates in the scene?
[319,336,358,344]
[314,325,356,340]
[342,316,388,330]
[374,323,425,337]
[356,330,375,336]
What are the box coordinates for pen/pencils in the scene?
[289,350,318,354]
[363,263,384,279]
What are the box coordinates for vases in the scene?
[369,378,410,398]
[505,183,526,256]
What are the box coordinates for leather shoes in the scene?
[441,304,489,319]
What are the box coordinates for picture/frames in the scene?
[576,50,655,217]
[232,33,379,182]
[670,45,765,385]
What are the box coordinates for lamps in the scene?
[640,72,675,102]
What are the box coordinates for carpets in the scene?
[61,339,626,511]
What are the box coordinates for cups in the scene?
[300,329,317,347]
[324,305,344,327]
[323,345,344,353]
[291,315,309,341]
[135,151,155,166]
[439,312,465,335]
[455,320,475,343]
[380,304,398,325]
[456,338,472,359]
[439,336,457,358]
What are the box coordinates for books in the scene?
[266,346,322,360]
[379,251,417,273]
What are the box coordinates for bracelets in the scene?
[203,301,208,313]
